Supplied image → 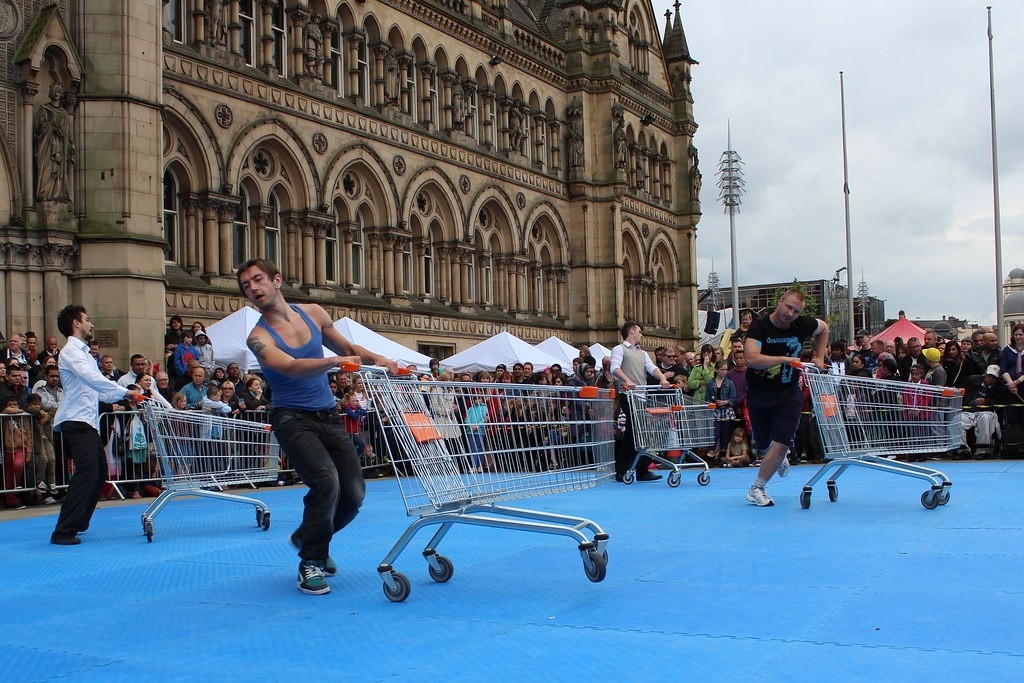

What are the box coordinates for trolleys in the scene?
[126,384,283,544]
[339,359,617,596]
[791,360,967,510]
[621,384,719,487]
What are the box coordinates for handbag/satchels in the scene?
[129,413,147,451]
[112,434,125,457]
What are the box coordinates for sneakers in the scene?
[296,557,331,595]
[776,447,789,478]
[48,483,58,495]
[38,481,48,493]
[744,484,775,507]
[44,496,57,505]
[289,530,337,577]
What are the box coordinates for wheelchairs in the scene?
[954,402,1006,461]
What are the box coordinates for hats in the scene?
[495,364,506,370]
[854,329,871,340]
[961,336,975,346]
[922,347,941,362]
[986,364,1001,378]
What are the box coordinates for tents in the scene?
[847,314,963,347]
[204,305,264,377]
[442,331,574,376]
[587,341,613,372]
[536,335,592,370]
[323,316,454,374]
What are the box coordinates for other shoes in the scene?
[636,470,663,481]
[954,451,972,460]
[50,533,81,544]
[8,502,27,510]
[974,453,988,460]
[791,452,828,465]
[706,449,717,458]
[748,459,763,466]
[615,472,627,482]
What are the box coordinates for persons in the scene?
[0,313,1024,509]
[235,258,399,594]
[614,119,626,169]
[744,288,830,506]
[453,74,465,130]
[609,322,671,483]
[303,11,324,79]
[51,304,140,545]
[690,159,702,203]
[35,81,76,203]
[209,0,225,47]
[510,100,523,147]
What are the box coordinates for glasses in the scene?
[663,355,676,358]
[223,388,233,390]
[21,377,27,379]
[702,349,713,354]
